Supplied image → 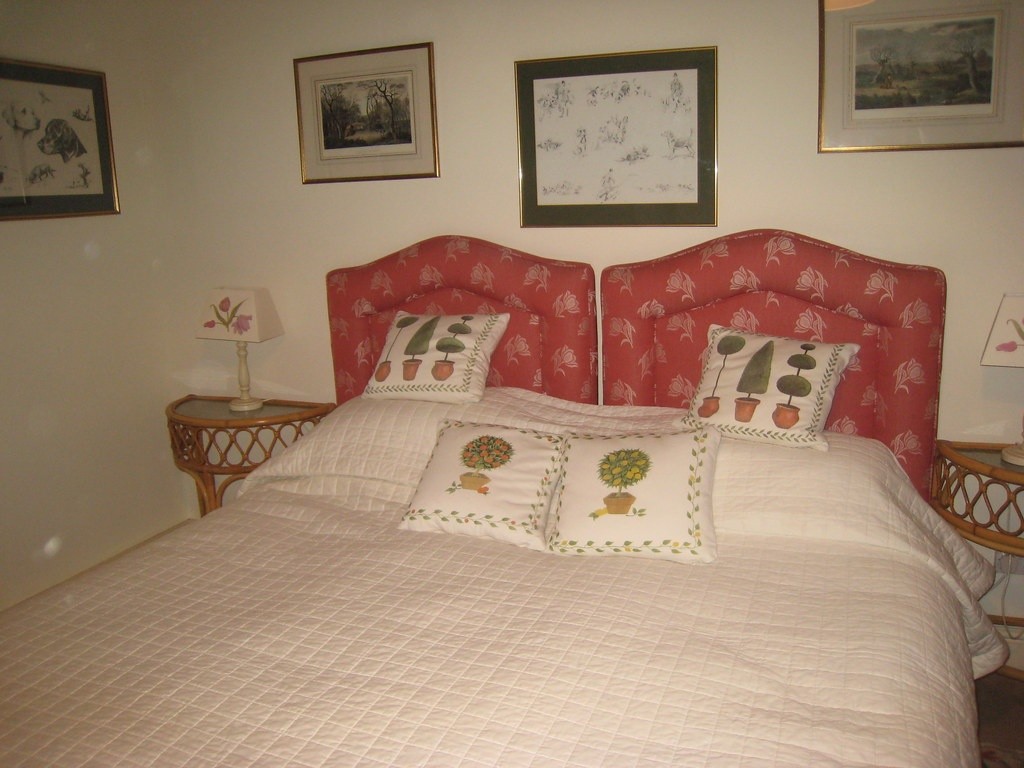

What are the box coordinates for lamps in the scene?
[194,287,284,412]
[978,295,1024,467]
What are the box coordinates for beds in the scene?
[0,234,982,768]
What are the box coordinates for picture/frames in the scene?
[514,46,718,229]
[0,58,121,221]
[817,0,1024,152]
[293,42,440,184]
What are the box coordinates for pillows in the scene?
[542,427,721,565]
[671,324,860,452]
[398,419,571,552]
[362,309,510,405]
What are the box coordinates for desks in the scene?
[926,441,1024,682]
[165,394,336,522]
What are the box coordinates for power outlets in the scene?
[994,546,1024,575]
